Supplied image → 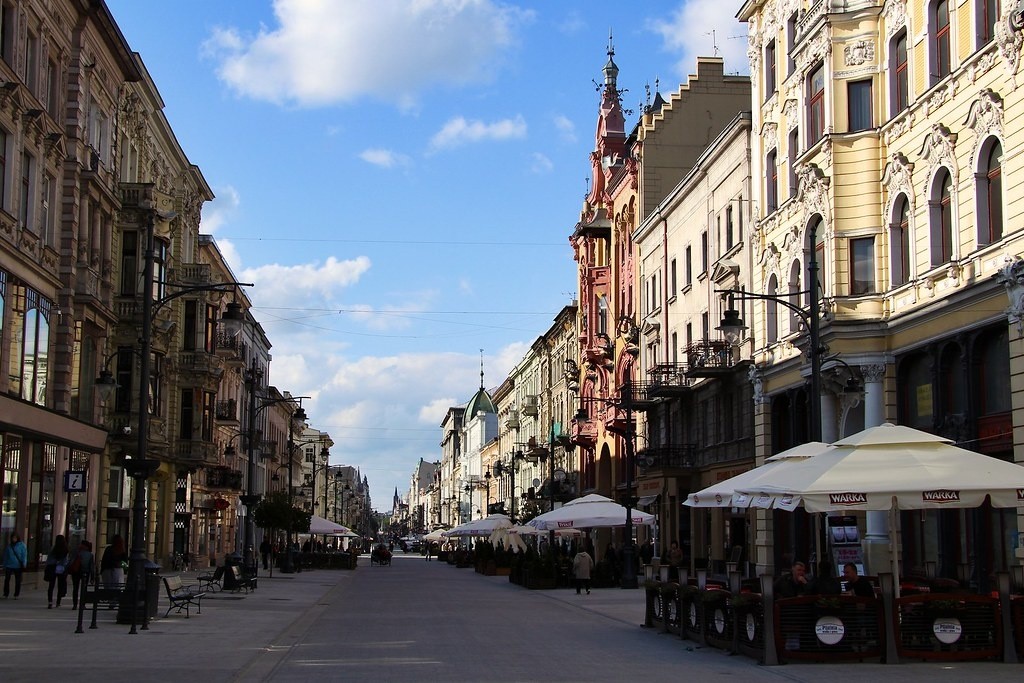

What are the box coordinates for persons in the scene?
[573,544,594,594]
[843,562,875,639]
[378,540,394,552]
[45,535,70,607]
[68,540,95,610]
[2,530,27,599]
[532,538,577,561]
[773,562,812,643]
[665,540,683,579]
[604,538,653,576]
[425,541,467,562]
[403,543,407,554]
[101,533,129,610]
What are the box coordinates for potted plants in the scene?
[921,598,962,624]
[643,579,660,597]
[661,581,673,602]
[813,596,845,618]
[700,591,722,609]
[732,593,753,617]
[677,584,701,606]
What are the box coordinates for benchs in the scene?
[196,566,224,594]
[163,575,206,619]
[85,583,128,611]
[227,566,258,595]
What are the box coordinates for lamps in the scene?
[48,303,62,315]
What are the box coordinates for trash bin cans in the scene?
[144,558,163,618]
[224,552,245,590]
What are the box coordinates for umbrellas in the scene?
[682,423,1024,642]
[441,513,528,552]
[507,493,657,542]
[298,515,360,551]
[260,535,331,570]
[423,529,460,541]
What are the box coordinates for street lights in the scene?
[515,416,571,556]
[439,463,502,528]
[222,357,311,580]
[714,225,871,444]
[314,462,363,528]
[93,207,255,625]
[271,412,330,573]
[484,446,528,526]
[574,395,658,590]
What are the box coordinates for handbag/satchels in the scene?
[44,564,56,581]
[68,559,80,574]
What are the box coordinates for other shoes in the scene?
[0,595,9,598]
[48,604,52,608]
[56,604,62,609]
[13,595,22,599]
[72,605,77,610]
[587,589,590,594]
